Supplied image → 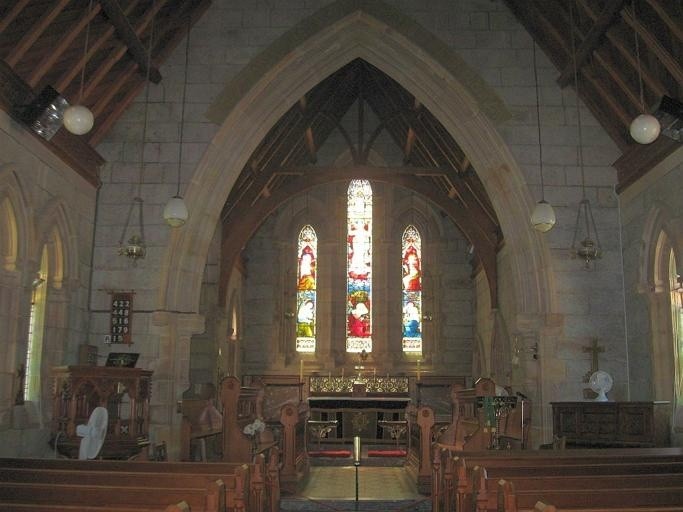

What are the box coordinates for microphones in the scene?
[516,392,528,399]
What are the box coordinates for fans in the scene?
[589,372,613,402]
[76,407,108,461]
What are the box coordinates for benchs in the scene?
[431,445,683,512]
[0,447,280,512]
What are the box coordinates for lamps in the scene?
[117,1,155,269]
[163,1,193,228]
[63,1,94,136]
[569,0,606,268]
[630,0,665,144]
[529,1,557,233]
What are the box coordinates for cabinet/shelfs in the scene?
[53,365,151,462]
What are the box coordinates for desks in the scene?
[539,400,674,449]
